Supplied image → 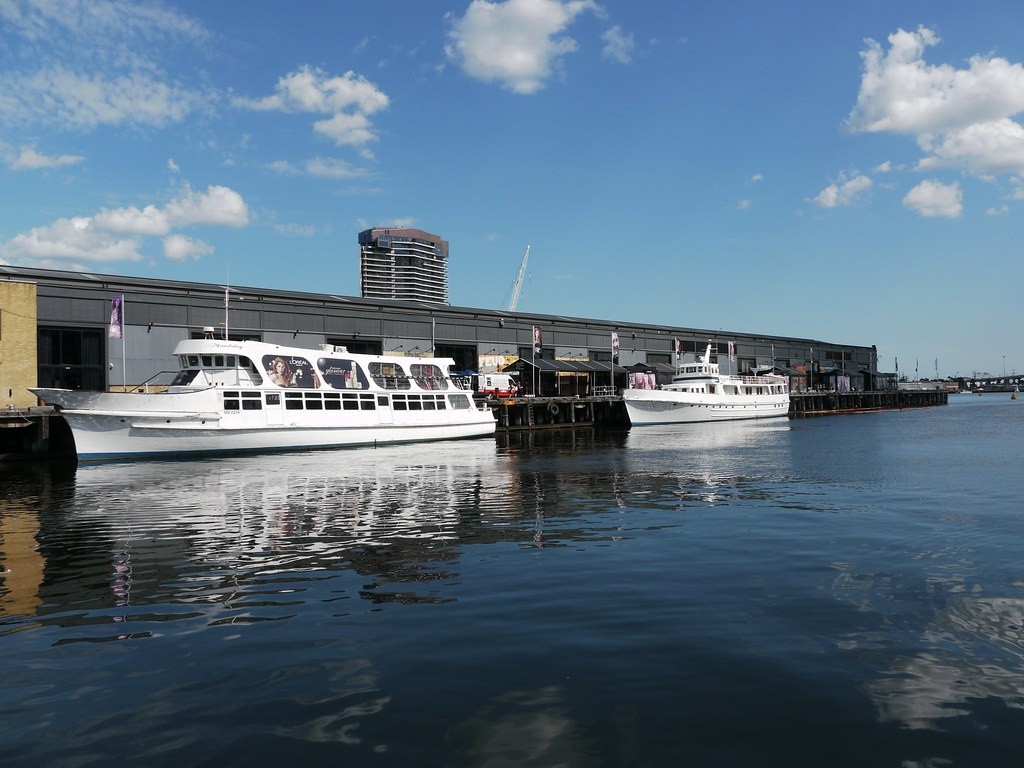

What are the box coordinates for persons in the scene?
[508,382,523,398]
[269,357,297,388]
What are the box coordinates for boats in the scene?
[623,338,791,425]
[25,326,500,452]
